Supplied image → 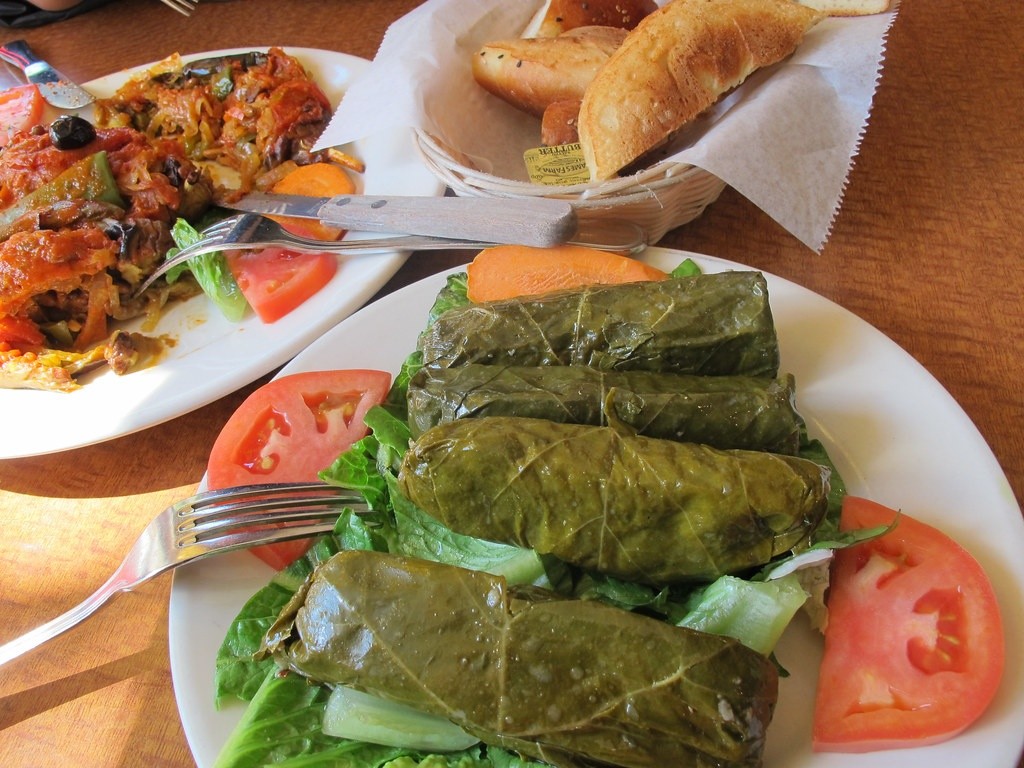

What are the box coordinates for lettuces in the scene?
[159,219,246,319]
[214,274,900,768]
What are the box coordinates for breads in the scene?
[473,0,892,182]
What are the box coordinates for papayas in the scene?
[462,246,677,303]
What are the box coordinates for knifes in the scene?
[214,184,579,250]
[3,38,98,111]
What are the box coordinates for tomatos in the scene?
[222,162,359,323]
[204,368,392,571]
[808,493,1005,751]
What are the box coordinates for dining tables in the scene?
[1,9,1024,768]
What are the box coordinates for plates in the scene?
[168,237,1022,768]
[0,43,423,457]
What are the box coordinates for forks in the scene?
[128,210,647,301]
[0,480,370,670]
